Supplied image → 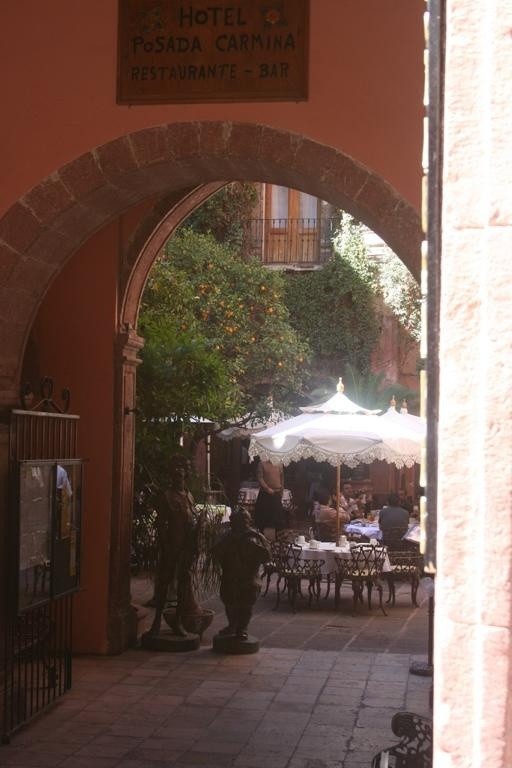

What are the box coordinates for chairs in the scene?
[262,523,423,619]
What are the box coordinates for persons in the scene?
[215,506,272,637]
[145,452,199,637]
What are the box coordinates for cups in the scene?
[309,540,319,548]
[295,536,305,544]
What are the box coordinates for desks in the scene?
[351,517,420,550]
[238,487,292,525]
[146,504,232,572]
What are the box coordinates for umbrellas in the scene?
[247,392,427,546]
[146,395,291,495]
